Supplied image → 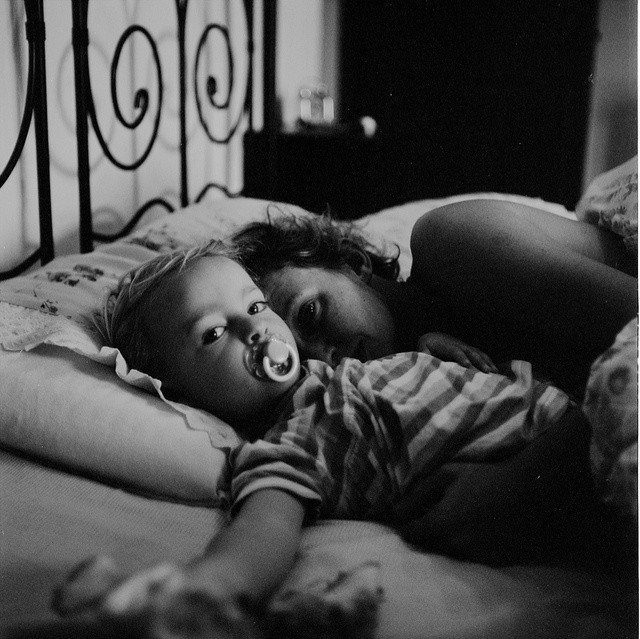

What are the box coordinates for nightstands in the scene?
[233,120,417,226]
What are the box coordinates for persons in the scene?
[88,236,638,638]
[224,197,639,404]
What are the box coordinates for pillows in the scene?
[2,197,325,509]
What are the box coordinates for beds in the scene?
[1,1,638,637]
